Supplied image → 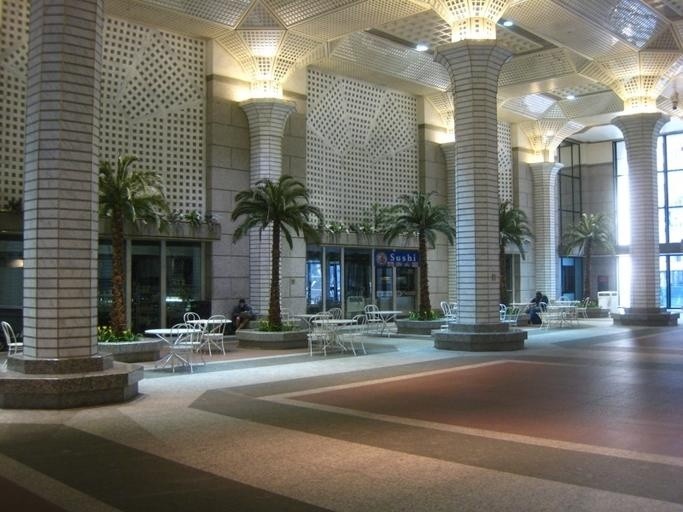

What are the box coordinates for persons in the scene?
[525,292,548,324]
[232,298,254,332]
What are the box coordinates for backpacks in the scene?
[239,311,253,319]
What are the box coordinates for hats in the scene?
[239,299,245,303]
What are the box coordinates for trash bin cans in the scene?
[598,291,617,308]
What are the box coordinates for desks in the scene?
[364,310,403,338]
[509,302,536,326]
[294,314,332,330]
[144,319,232,370]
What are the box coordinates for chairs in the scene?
[0,321,24,369]
[527,296,590,329]
[164,312,226,373]
[303,304,383,358]
[499,303,521,331]
[440,301,458,329]
